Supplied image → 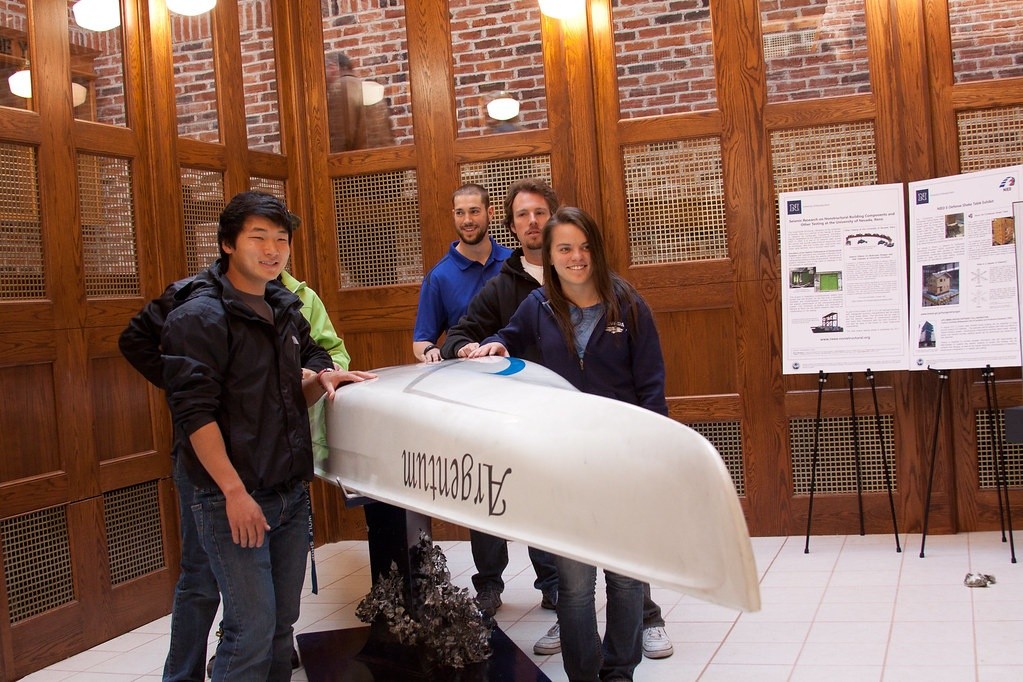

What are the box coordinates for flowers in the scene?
[353,528,495,670]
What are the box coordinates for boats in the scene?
[810,312,843,333]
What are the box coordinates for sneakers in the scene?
[540,597,557,609]
[532,624,562,654]
[641,626,673,658]
[475,590,502,616]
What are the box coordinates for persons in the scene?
[119,191,378,682]
[441,179,672,659]
[324,51,404,287]
[413,183,558,614]
[470,207,669,682]
[482,94,535,206]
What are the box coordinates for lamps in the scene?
[72,82,87,108]
[8,62,31,99]
[166,1,216,16]
[362,80,383,106]
[485,91,519,120]
[72,0,120,31]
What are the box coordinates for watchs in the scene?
[424,345,439,355]
[317,368,335,390]
